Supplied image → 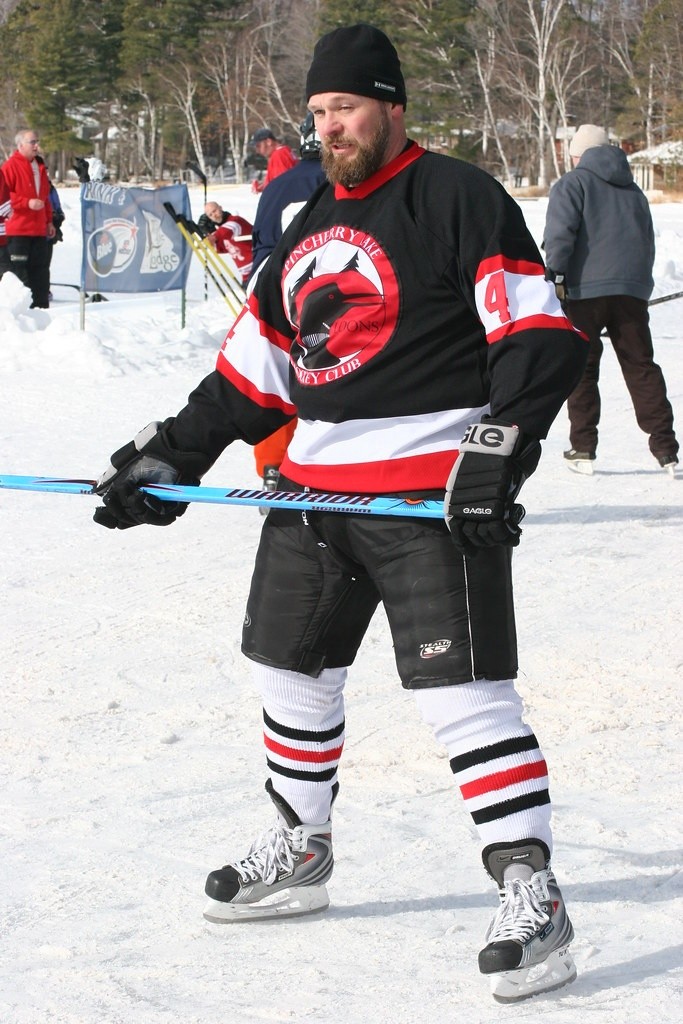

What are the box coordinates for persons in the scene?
[95,23,590,1001]
[0,130,66,309]
[206,201,253,291]
[541,124,679,479]
[253,129,297,194]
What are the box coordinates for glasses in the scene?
[21,140,41,146]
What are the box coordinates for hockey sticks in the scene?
[50,279,81,298]
[598,288,683,338]
[0,473,526,526]
[184,159,211,302]
[162,199,248,318]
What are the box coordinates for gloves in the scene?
[545,267,568,313]
[93,417,214,530]
[444,414,542,556]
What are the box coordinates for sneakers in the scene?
[658,456,678,479]
[564,449,596,476]
[201,776,340,924]
[478,837,578,1005]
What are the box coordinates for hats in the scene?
[306,23,407,115]
[248,127,272,146]
[568,123,610,157]
